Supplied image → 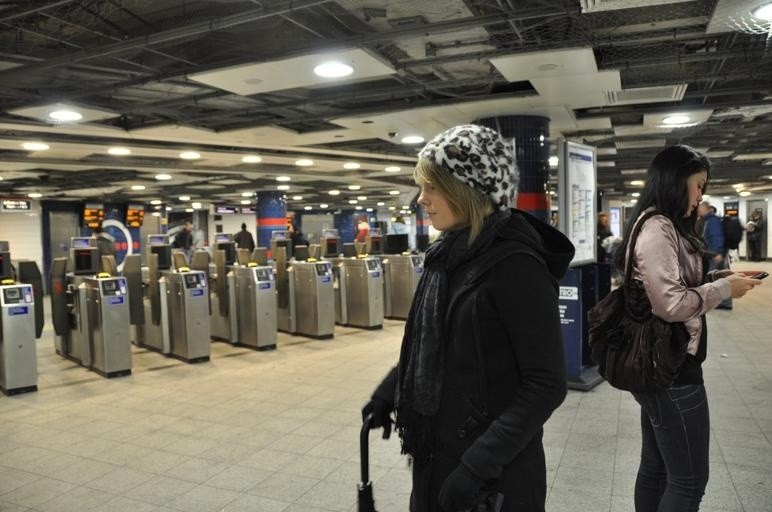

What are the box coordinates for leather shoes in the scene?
[714,304,731,309]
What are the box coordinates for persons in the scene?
[234,223,255,255]
[697,193,763,312]
[290,224,310,251]
[173,221,194,252]
[587,141,770,512]
[597,211,614,260]
[359,121,579,512]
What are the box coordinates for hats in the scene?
[417,123,520,213]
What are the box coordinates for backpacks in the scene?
[718,216,742,249]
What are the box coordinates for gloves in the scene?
[362,396,389,428]
[438,464,475,512]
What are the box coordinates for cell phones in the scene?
[751,272,769,280]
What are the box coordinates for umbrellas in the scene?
[358,412,393,512]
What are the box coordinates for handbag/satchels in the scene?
[586,277,690,394]
